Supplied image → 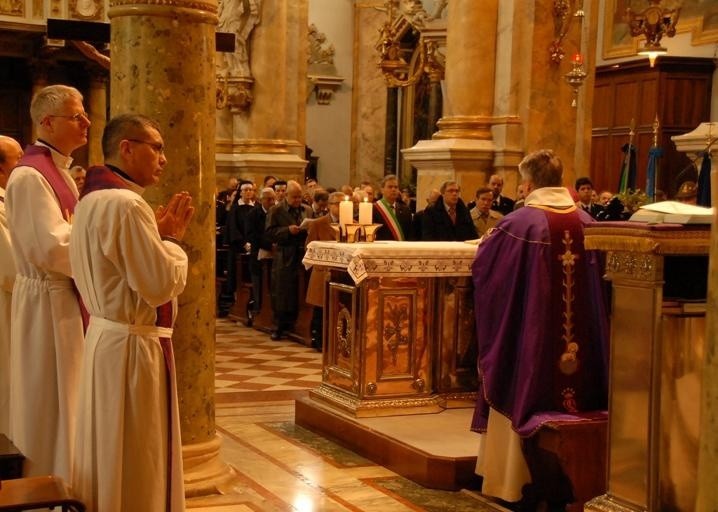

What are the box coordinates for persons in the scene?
[599,190,612,207]
[412,175,514,242]
[514,181,524,210]
[67,112,197,512]
[69,165,87,195]
[471,146,613,511]
[216,173,415,350]
[7,85,92,480]
[1,133,24,444]
[574,177,607,219]
[589,189,599,203]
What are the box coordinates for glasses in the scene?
[328,202,340,207]
[117,138,169,153]
[445,188,460,194]
[39,112,89,126]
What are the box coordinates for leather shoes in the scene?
[271,328,280,339]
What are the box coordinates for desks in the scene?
[0,432,25,480]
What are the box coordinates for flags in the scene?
[645,146,662,202]
[695,152,712,208]
[617,143,637,195]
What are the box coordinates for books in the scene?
[628,200,714,225]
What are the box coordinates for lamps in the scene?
[566,46,587,107]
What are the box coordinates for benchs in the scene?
[216,253,317,347]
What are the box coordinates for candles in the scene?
[358,197,373,236]
[338,195,354,236]
